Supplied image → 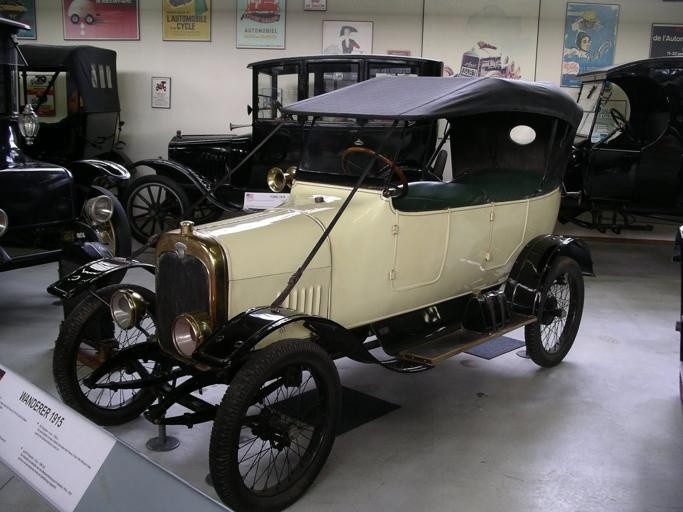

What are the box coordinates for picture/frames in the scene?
[236,0,285,49]
[592,101,627,142]
[162,0,211,42]
[24,76,54,116]
[422,1,540,80]
[305,0,327,11]
[152,77,171,108]
[1,0,36,39]
[322,20,372,55]
[649,24,683,57]
[62,0,140,41]
[561,2,619,88]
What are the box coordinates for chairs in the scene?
[464,169,546,204]
[381,180,486,213]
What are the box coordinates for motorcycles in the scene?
[155,81,167,92]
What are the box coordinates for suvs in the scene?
[48,75,596,512]
[0,17,131,289]
[672,223,683,406]
[124,56,444,249]
[442,40,522,78]
[556,55,682,234]
[8,44,136,219]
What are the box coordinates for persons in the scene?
[575,32,592,60]
